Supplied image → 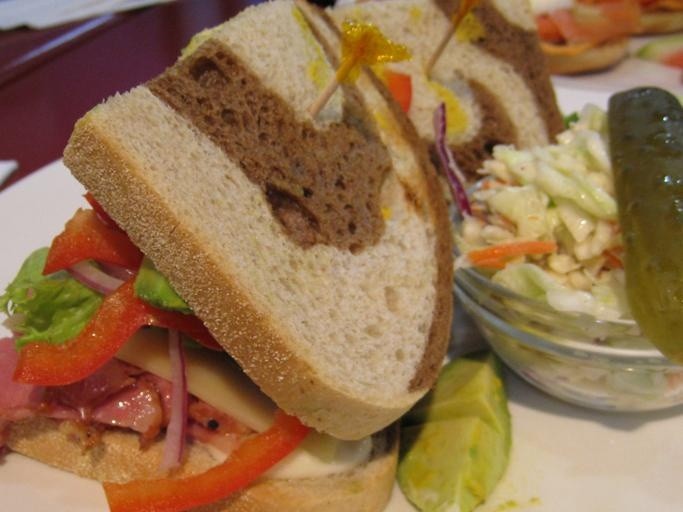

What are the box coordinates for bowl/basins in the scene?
[448,171,681,415]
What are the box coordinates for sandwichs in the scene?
[0,0,683,512]
